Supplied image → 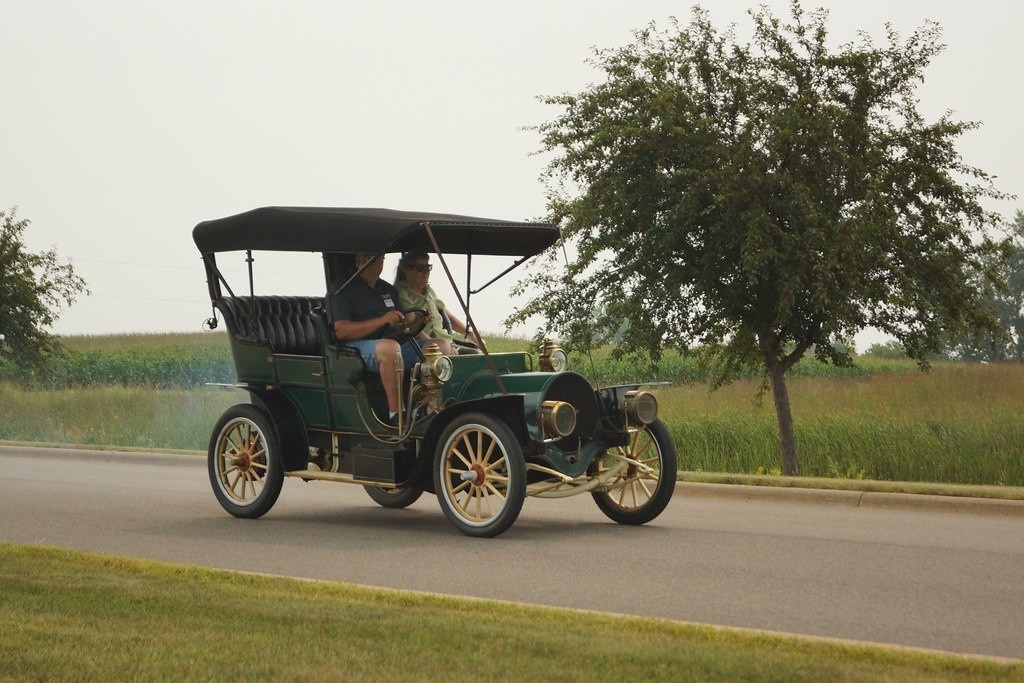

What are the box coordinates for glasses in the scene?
[407,264,433,272]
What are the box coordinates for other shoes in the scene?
[386,412,413,427]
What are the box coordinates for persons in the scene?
[394,251,488,357]
[325,256,451,425]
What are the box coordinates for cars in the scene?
[191,205,680,538]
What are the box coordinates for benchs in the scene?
[309,307,412,390]
[222,296,335,355]
